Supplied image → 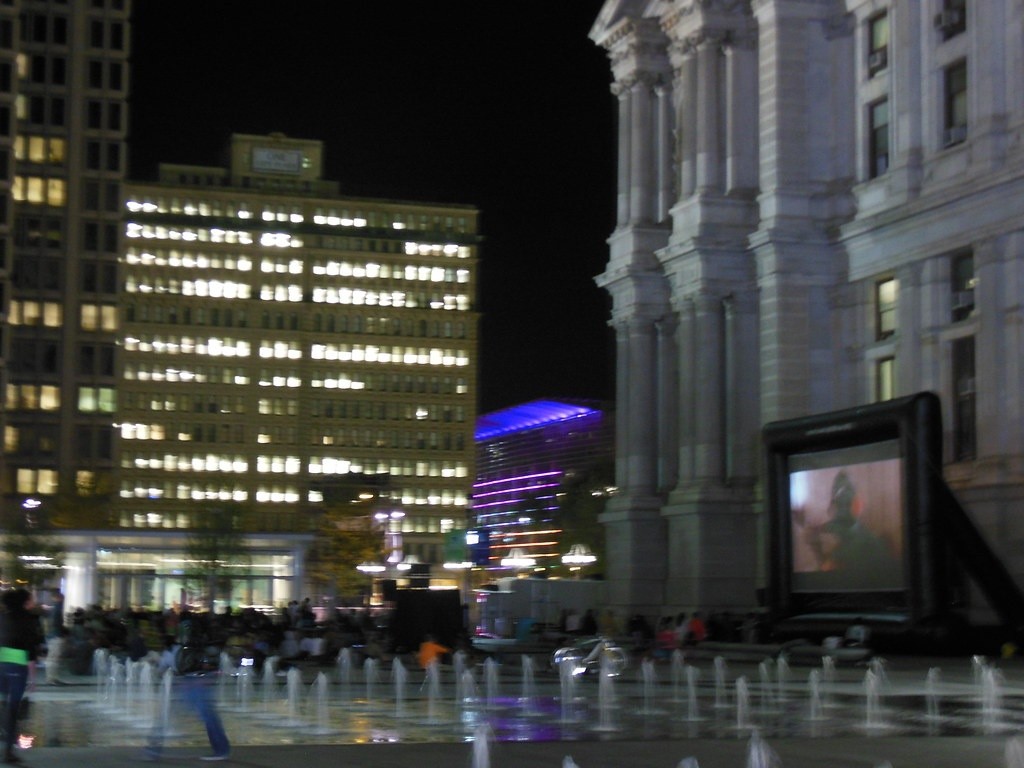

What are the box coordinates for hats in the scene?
[187,601,211,614]
[829,472,848,499]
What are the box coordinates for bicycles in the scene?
[550,636,629,679]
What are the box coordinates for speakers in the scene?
[756,588,766,600]
[382,579,396,601]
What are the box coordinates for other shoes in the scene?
[129,751,161,761]
[198,754,230,761]
[49,680,64,686]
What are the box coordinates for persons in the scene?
[559,609,707,663]
[806,473,881,574]
[0,585,46,765]
[49,591,386,683]
[415,633,449,695]
